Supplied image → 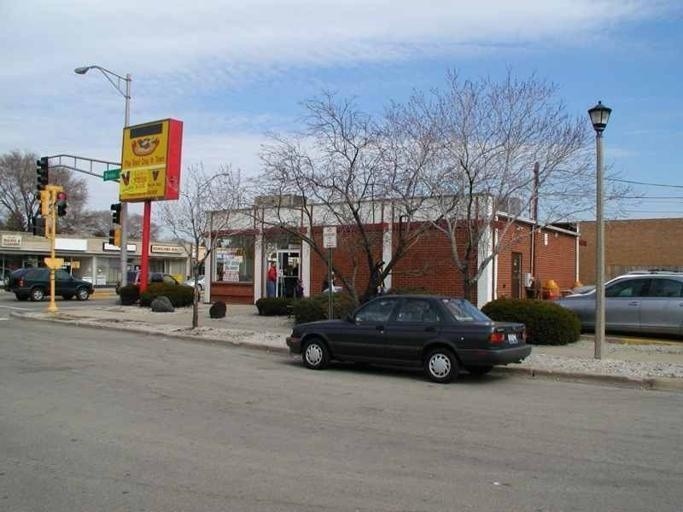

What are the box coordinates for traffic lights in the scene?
[108,229,120,247]
[32,217,45,237]
[37,191,47,215]
[110,203,121,224]
[36,159,46,190]
[58,192,66,217]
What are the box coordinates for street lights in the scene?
[588,102,612,358]
[74,65,130,286]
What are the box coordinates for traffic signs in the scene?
[322,226,337,248]
[103,168,120,181]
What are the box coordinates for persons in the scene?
[321,272,335,292]
[292,280,303,300]
[266,261,276,297]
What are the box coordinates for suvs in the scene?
[9,268,95,302]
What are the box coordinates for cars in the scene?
[287,293,531,383]
[554,274,682,334]
[116,270,180,295]
[183,274,205,291]
